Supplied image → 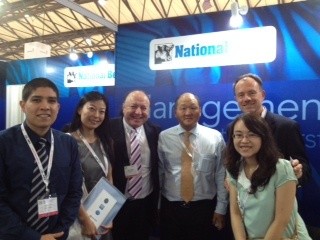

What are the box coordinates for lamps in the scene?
[230,2,248,28]
[69,46,78,60]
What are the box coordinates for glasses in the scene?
[232,133,263,139]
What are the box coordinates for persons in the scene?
[0,77,84,240]
[222,73,314,240]
[62,91,114,240]
[158,91,233,240]
[108,88,162,240]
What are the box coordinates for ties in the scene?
[181,132,195,204]
[128,129,143,199]
[28,137,49,234]
